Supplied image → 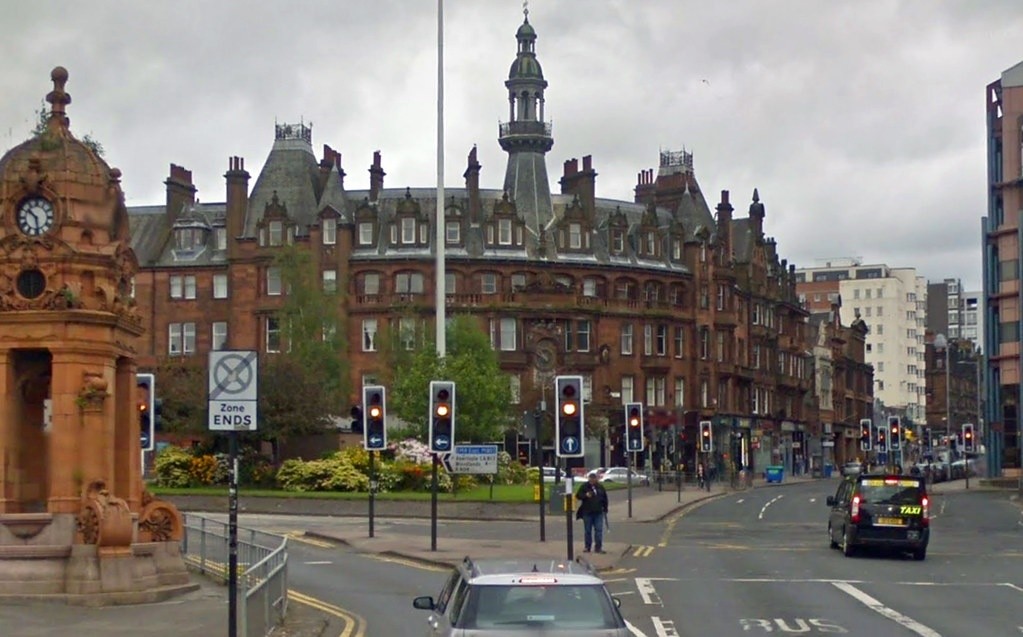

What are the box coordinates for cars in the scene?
[826,473,930,560]
[583,466,653,488]
[525,467,589,484]
[842,463,864,477]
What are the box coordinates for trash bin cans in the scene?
[823,463,832,478]
[765,466,784,483]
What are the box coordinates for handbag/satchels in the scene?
[576,506,583,519]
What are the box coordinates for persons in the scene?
[698,463,708,488]
[911,461,921,477]
[895,460,903,475]
[576,473,609,553]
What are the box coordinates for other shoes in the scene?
[583,549,590,552]
[595,551,606,554]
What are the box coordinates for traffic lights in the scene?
[625,403,644,451]
[962,424,974,452]
[860,419,872,450]
[878,426,888,453]
[137,374,154,450]
[428,381,455,453]
[556,376,584,457]
[363,386,387,450]
[888,416,901,451]
[700,421,713,452]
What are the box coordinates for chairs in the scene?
[540,584,578,618]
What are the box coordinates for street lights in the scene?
[934,333,952,482]
[926,428,931,451]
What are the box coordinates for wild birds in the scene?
[700,79,709,85]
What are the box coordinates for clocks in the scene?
[17,198,54,236]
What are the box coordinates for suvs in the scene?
[412,555,632,637]
